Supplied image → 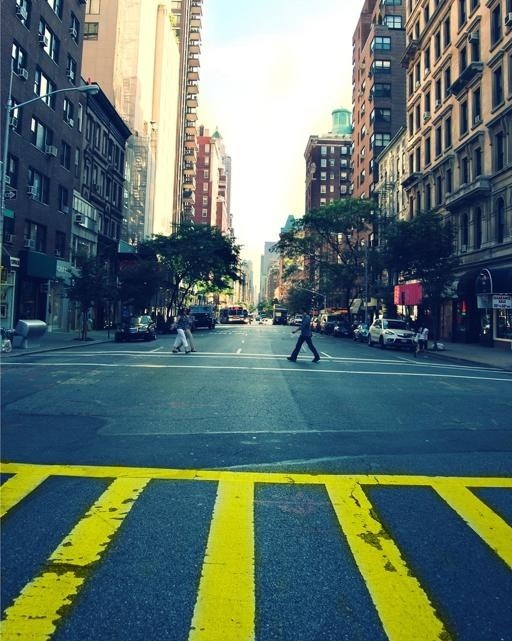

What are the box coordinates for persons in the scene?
[177,306,198,352]
[287,306,320,363]
[415,323,429,358]
[170,308,190,354]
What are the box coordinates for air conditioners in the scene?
[504,12,512,27]
[475,114,480,125]
[423,111,430,121]
[0,28,85,257]
[93,184,101,192]
[359,72,372,163]
[467,32,479,43]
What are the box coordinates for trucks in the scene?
[184,303,249,331]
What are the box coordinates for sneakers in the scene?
[287,357,296,362]
[312,357,320,362]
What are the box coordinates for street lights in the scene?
[349,235,369,323]
[476,268,497,346]
[0,84,123,282]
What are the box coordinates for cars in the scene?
[251,304,418,353]
[112,315,160,344]
[164,315,198,333]
[491,314,511,329]
